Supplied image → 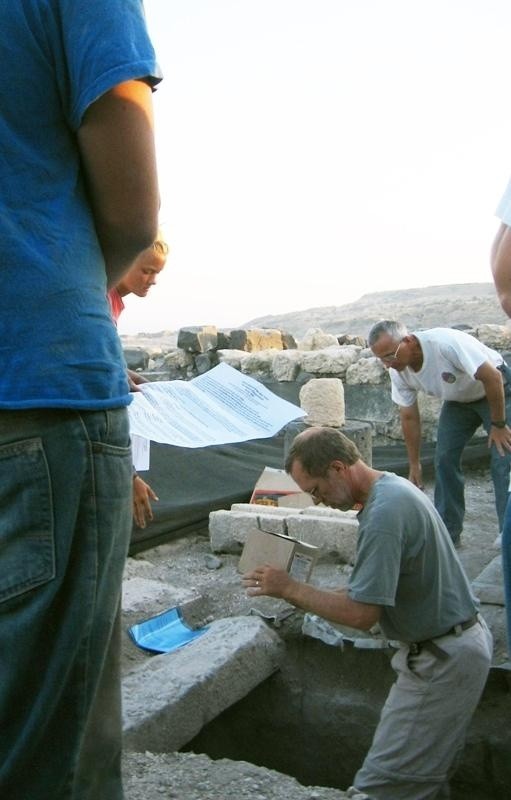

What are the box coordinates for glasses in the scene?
[375,341,402,363]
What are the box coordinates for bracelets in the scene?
[132,465,137,479]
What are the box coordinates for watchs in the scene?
[491,417,508,428]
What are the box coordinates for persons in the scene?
[2,0,169,800]
[107,239,168,530]
[490,180,511,657]
[369,320,511,547]
[240,425,495,800]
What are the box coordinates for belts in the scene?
[400,612,482,648]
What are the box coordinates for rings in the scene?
[256,581,259,587]
